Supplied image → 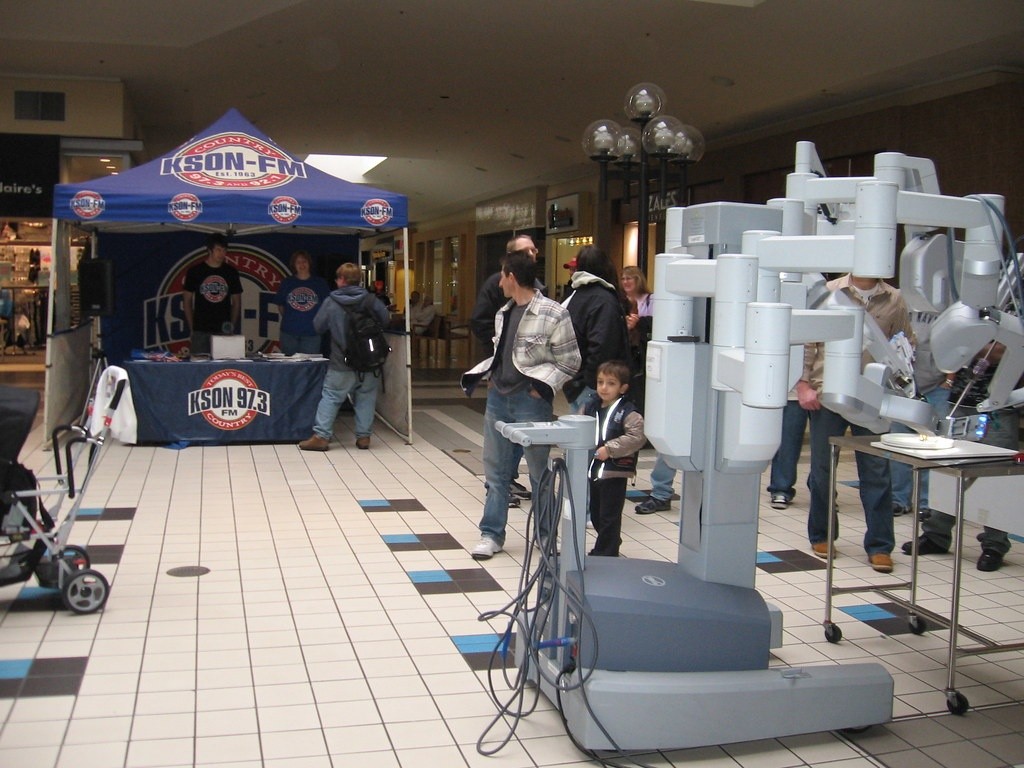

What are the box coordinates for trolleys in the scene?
[823,434,1023,715]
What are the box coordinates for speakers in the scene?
[79,256,117,320]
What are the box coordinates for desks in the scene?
[116,356,330,445]
[827,433,1024,696]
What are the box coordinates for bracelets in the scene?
[946,379,954,386]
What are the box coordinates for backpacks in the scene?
[336,293,390,373]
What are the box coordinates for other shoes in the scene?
[585,521,594,530]
[893,502,904,516]
[299,434,330,451]
[867,553,891,571]
[356,437,370,449]
[812,540,835,559]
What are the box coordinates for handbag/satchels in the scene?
[0,459,55,536]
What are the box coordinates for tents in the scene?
[43,107,414,450]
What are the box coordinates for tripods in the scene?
[77,315,109,428]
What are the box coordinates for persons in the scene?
[182,231,243,357]
[577,358,647,557]
[635,450,676,514]
[555,243,656,525]
[797,275,918,571]
[474,234,547,508]
[271,249,331,357]
[391,291,436,335]
[471,252,582,558]
[890,287,955,521]
[902,342,1017,574]
[299,263,390,451]
[766,383,845,511]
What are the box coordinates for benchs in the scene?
[393,314,472,369]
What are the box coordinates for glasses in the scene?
[526,249,538,254]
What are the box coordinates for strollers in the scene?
[3,305,35,355]
[0,365,128,614]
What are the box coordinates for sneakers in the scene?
[471,535,502,558]
[485,489,521,507]
[771,494,786,508]
[534,536,561,554]
[507,479,533,500]
[978,547,1000,571]
[634,494,671,514]
[901,534,948,555]
